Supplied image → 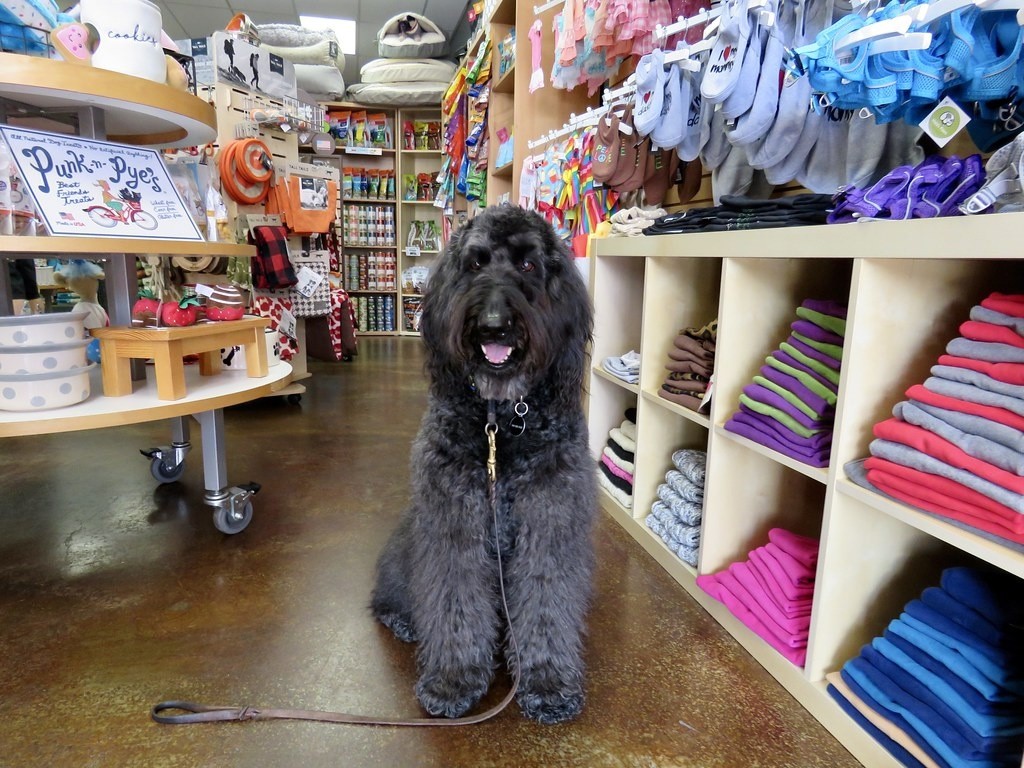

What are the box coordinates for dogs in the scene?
[365,195,597,731]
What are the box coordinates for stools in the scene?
[88,315,271,400]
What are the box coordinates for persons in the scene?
[251,54,259,88]
[227,39,235,71]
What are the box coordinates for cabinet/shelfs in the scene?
[0,0,1024,768]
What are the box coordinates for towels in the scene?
[651,500,700,548]
[645,514,699,567]
[657,318,718,415]
[842,457,1024,555]
[696,527,818,667]
[723,299,848,468]
[601,349,643,385]
[863,292,1024,545]
[597,407,637,509]
[656,448,706,526]
[823,568,1024,768]
